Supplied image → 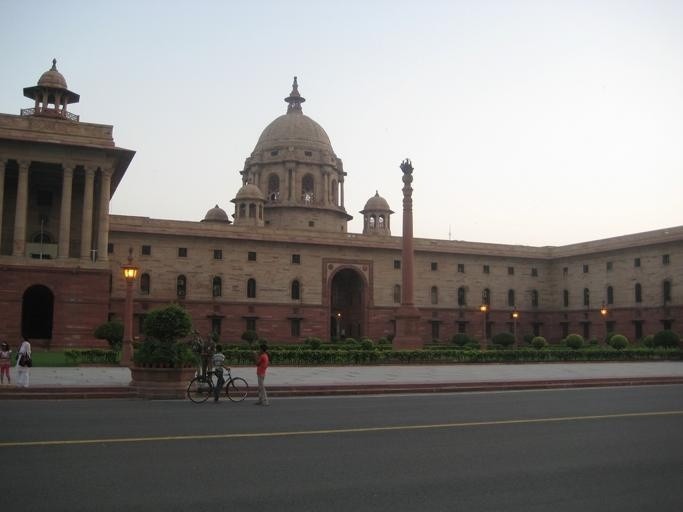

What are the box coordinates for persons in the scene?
[191,329,204,382]
[250,344,271,406]
[214,345,230,404]
[200,332,217,384]
[0,340,12,384]
[14,334,32,388]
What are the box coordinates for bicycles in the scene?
[187,368,248,403]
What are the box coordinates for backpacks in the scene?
[19,356,32,367]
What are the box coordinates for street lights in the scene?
[601,306,608,340]
[480,304,487,346]
[334,312,342,340]
[119,257,138,368]
[512,307,519,346]
